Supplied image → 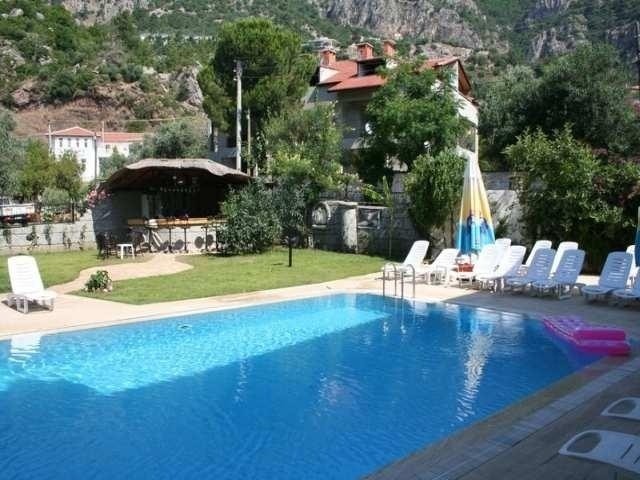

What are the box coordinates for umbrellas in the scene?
[455,152,496,264]
[96,157,255,218]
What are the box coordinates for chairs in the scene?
[96,229,145,260]
[379,237,640,309]
[5,255,59,314]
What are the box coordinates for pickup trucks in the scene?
[0,196,35,227]
